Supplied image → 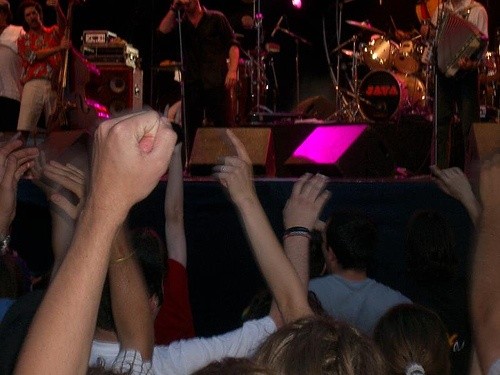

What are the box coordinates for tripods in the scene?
[246,28,274,113]
[328,39,365,121]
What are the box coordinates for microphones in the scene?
[271,17,283,36]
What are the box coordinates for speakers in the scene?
[148,67,183,118]
[186,127,281,179]
[83,63,143,118]
[463,123,499,177]
[282,123,384,180]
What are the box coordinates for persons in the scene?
[42,160,156,375]
[158,0,240,174]
[241,15,253,30]
[474,160,500,375]
[217,130,481,375]
[149,141,196,345]
[0,140,39,252]
[0,0,27,134]
[430,0,488,163]
[79,174,330,375]
[19,0,71,134]
[15,112,390,375]
[306,212,414,342]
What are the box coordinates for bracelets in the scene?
[0,235,11,251]
[108,250,137,264]
[282,227,312,241]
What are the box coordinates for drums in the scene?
[355,69,427,125]
[232,58,265,103]
[391,39,422,74]
[247,86,278,119]
[479,51,500,83]
[362,34,398,72]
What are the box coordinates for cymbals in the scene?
[341,49,364,61]
[342,19,381,31]
